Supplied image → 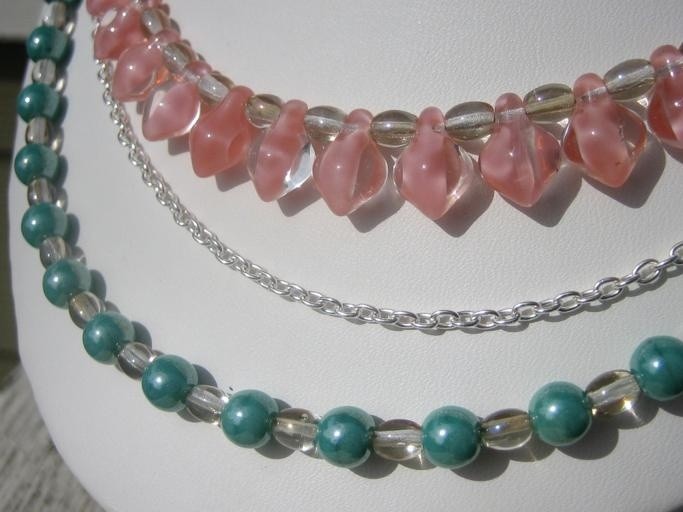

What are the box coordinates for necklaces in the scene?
[85,16,682,334]
[84,0,683,224]
[12,0,683,473]
